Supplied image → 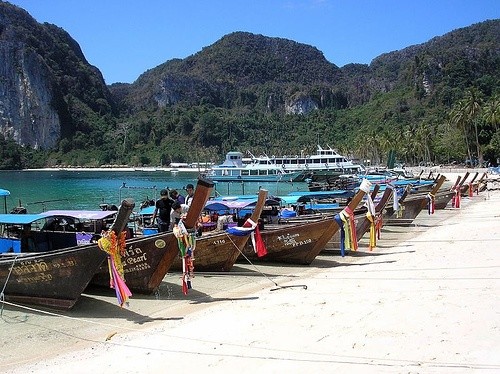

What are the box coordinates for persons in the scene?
[169,189,185,204]
[185,183,194,206]
[170,202,190,232]
[153,189,175,233]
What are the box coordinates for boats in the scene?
[27,189,268,272]
[140,178,372,265]
[290,175,446,225]
[6,178,215,295]
[0,188,135,310]
[287,184,413,228]
[215,188,393,248]
[416,171,486,208]
[204,183,380,255]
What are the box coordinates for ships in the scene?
[208,144,363,180]
